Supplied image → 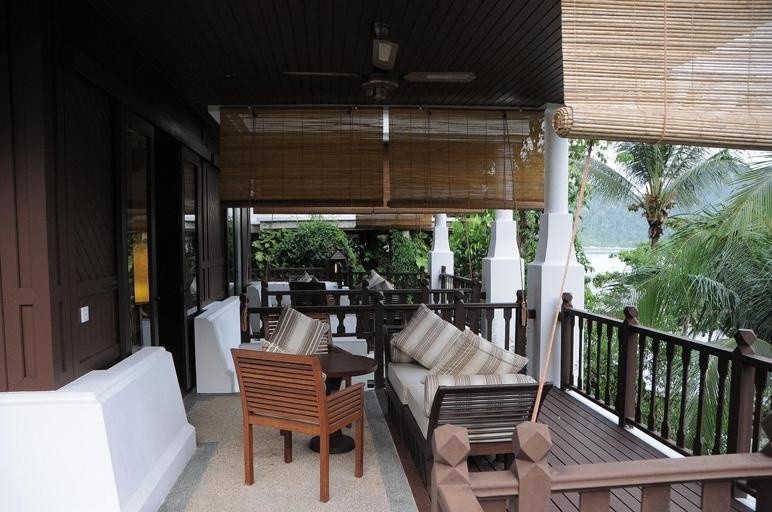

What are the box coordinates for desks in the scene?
[306,351,377,454]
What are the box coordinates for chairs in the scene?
[230,346,367,504]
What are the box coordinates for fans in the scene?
[281,20,479,102]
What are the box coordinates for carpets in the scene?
[157,384,421,512]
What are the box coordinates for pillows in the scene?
[365,268,384,287]
[430,329,528,377]
[391,304,459,369]
[424,373,536,419]
[296,271,312,283]
[270,306,328,354]
[259,340,327,388]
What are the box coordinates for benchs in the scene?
[384,320,554,471]
